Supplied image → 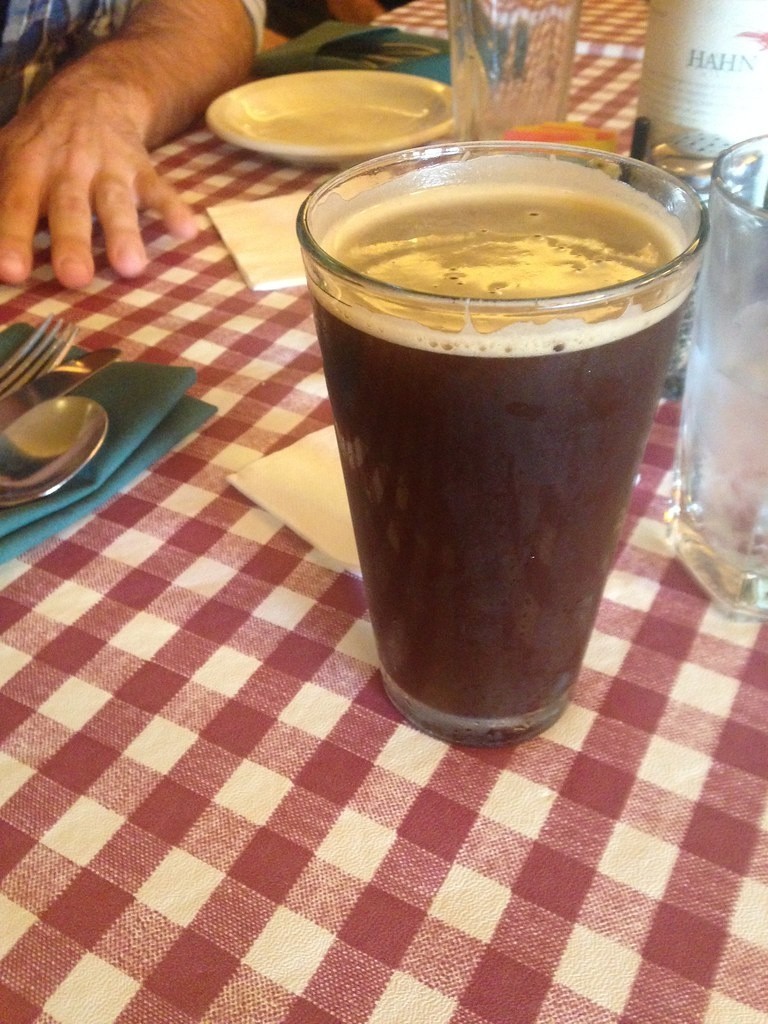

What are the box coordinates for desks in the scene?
[0,0,768,1024]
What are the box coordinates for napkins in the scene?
[224,423,362,577]
[207,189,311,292]
[0,324,219,566]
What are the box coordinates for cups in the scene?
[660,135,767,618]
[450,1,584,143]
[296,142,709,749]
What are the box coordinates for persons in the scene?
[258,0,411,51]
[0,0,267,289]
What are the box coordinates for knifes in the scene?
[0,348,123,432]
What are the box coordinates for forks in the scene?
[0,318,76,397]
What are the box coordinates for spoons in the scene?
[1,395,111,507]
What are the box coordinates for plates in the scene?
[203,69,451,167]
